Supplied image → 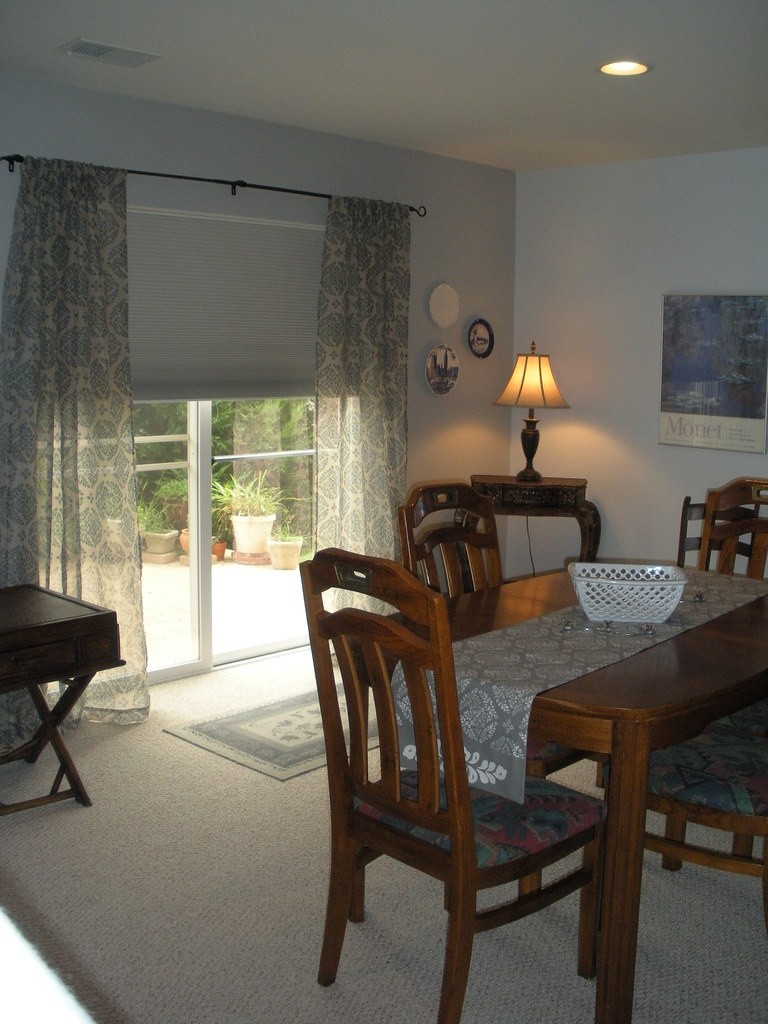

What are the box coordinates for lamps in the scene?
[491,341,571,482]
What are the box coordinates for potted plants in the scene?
[180,513,189,555]
[265,520,314,569]
[211,468,300,553]
[145,507,178,553]
[149,478,188,529]
[211,534,227,562]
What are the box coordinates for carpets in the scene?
[161,680,380,782]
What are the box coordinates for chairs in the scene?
[599,701,768,933]
[695,477,768,580]
[299,546,609,1024]
[397,481,504,599]
[677,495,760,569]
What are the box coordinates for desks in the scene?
[451,475,602,594]
[343,560,768,1024]
[0,583,126,817]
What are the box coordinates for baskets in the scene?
[567,561,689,624]
